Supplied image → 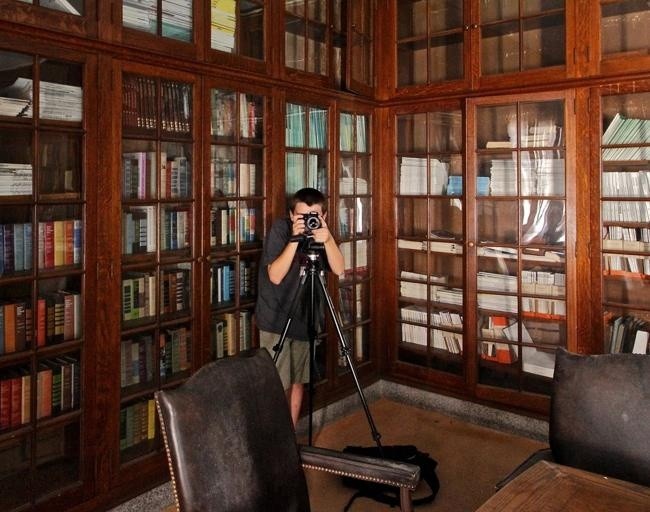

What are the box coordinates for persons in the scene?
[256,188,343,429]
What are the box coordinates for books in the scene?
[210,87,363,365]
[599,113,650,356]
[41,1,236,54]
[399,124,569,379]
[1,71,191,450]
[284,0,342,79]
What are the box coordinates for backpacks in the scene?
[340,444,440,511]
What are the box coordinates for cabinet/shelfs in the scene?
[0,0,650,512]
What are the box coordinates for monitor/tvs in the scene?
[272,236,381,449]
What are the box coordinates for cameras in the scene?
[303,212,322,235]
